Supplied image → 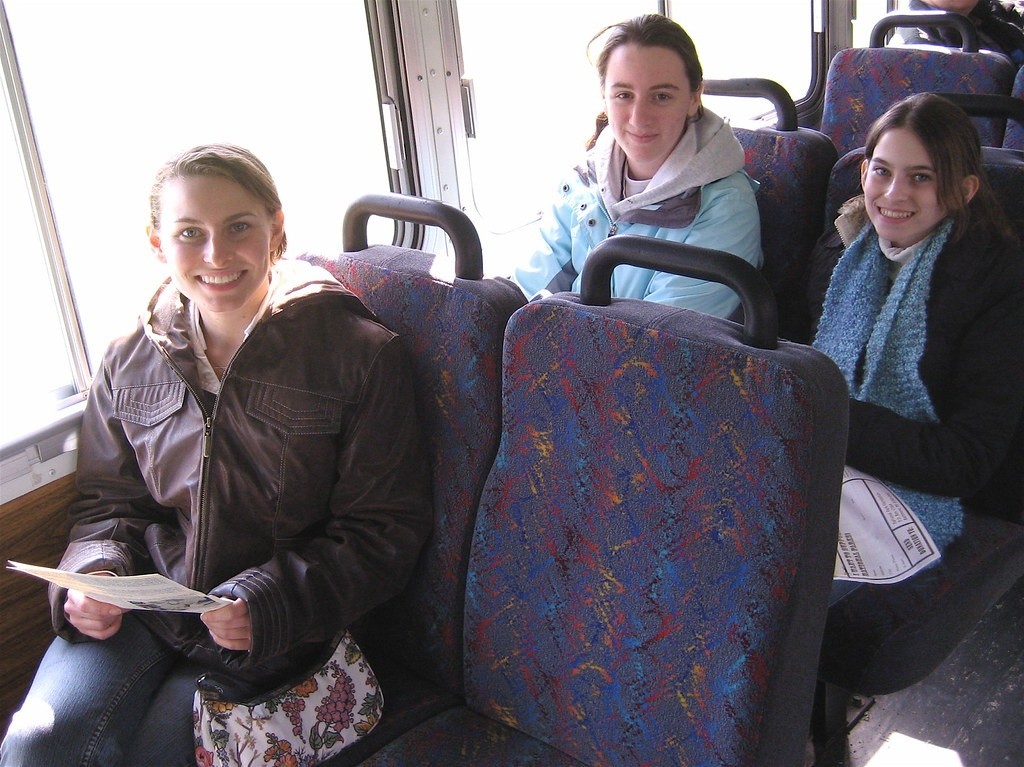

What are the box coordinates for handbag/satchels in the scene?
[192,628,383,767]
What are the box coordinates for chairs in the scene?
[584,77,839,342]
[294,192,529,767]
[360,234,848,767]
[810,93,1024,767]
[819,10,1015,156]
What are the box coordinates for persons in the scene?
[0,143,434,767]
[510,14,763,320]
[775,93,1024,608]
[888,0,1024,60]
